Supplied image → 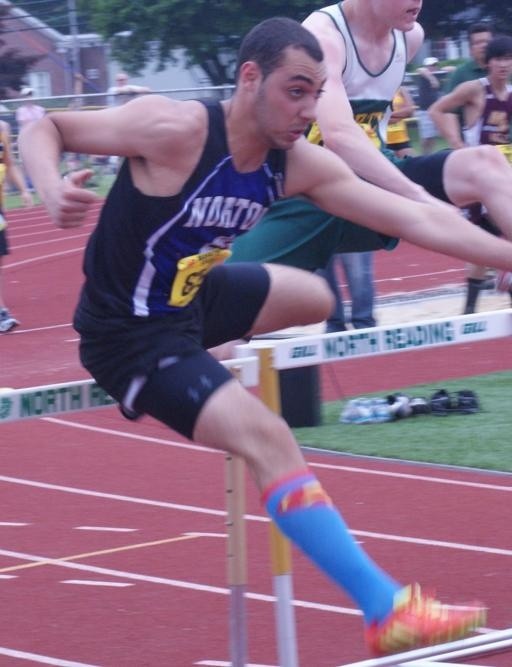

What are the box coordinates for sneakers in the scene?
[364,581,486,656]
[0,308,22,332]
[341,390,479,425]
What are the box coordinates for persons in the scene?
[226,1,512,335]
[64,73,153,176]
[0,89,46,333]
[18,18,512,656]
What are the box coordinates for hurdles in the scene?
[0,307,512,667]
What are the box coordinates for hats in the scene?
[20,87,34,95]
